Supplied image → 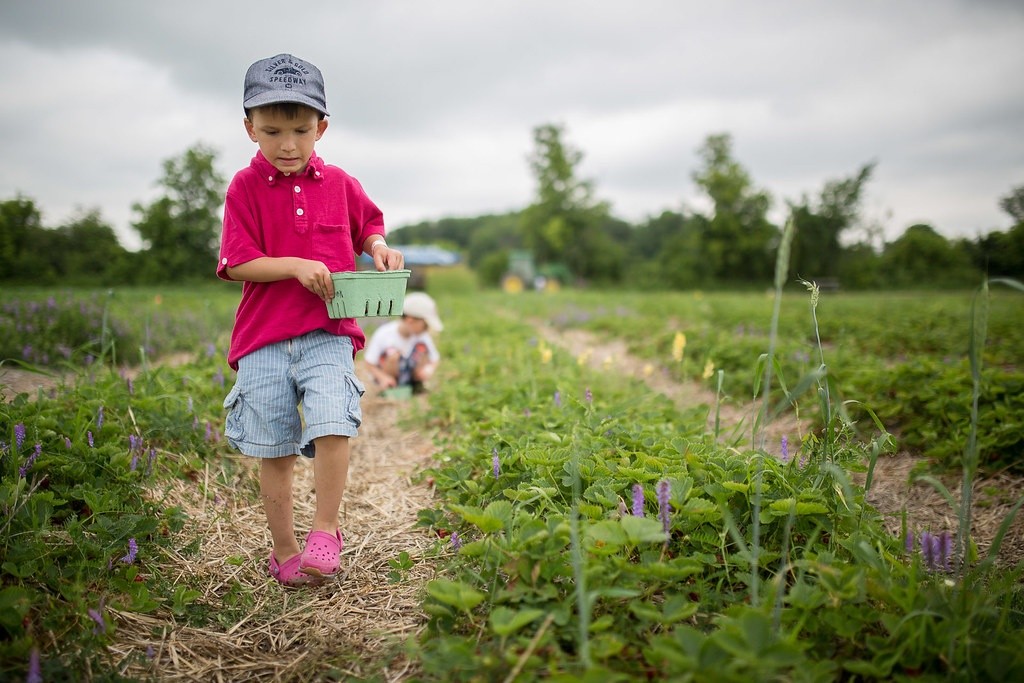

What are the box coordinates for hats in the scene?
[242,53,330,120]
[402,291,443,333]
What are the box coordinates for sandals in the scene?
[270,553,322,587]
[299,529,342,577]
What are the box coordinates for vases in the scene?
[325,269,411,319]
[384,386,413,403]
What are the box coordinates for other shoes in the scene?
[411,379,426,394]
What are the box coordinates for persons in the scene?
[217,53,405,589]
[366,292,443,400]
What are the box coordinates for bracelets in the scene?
[371,240,387,257]
